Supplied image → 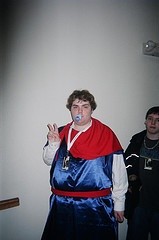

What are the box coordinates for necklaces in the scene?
[143,133,159,149]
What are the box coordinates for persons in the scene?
[123,106,159,240]
[41,89,129,240]
